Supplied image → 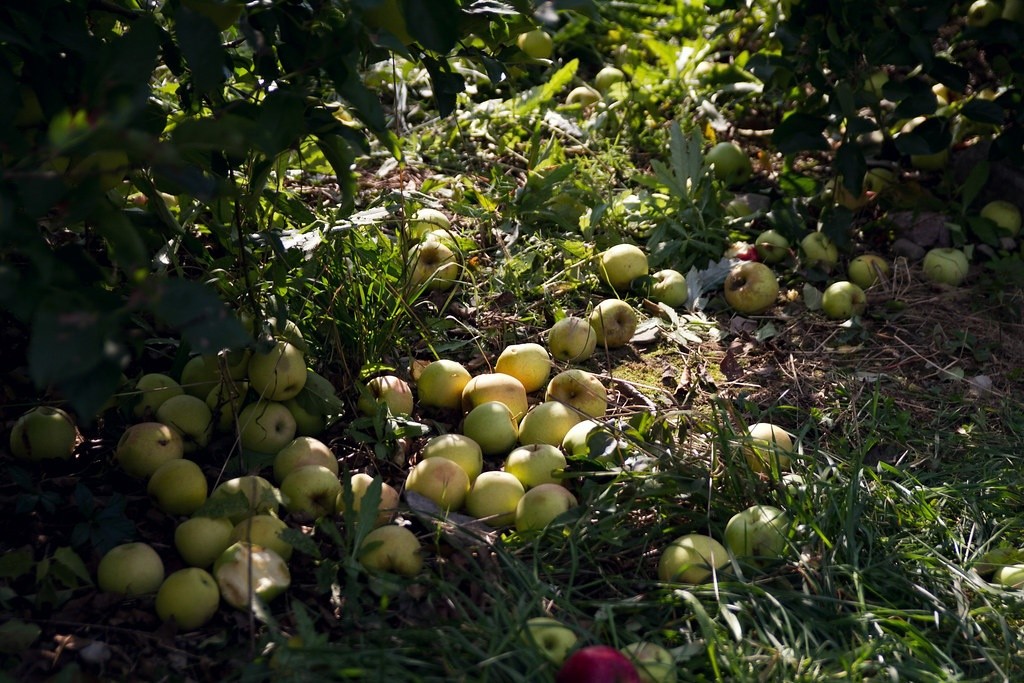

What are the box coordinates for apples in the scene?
[0,30,1024,683]
